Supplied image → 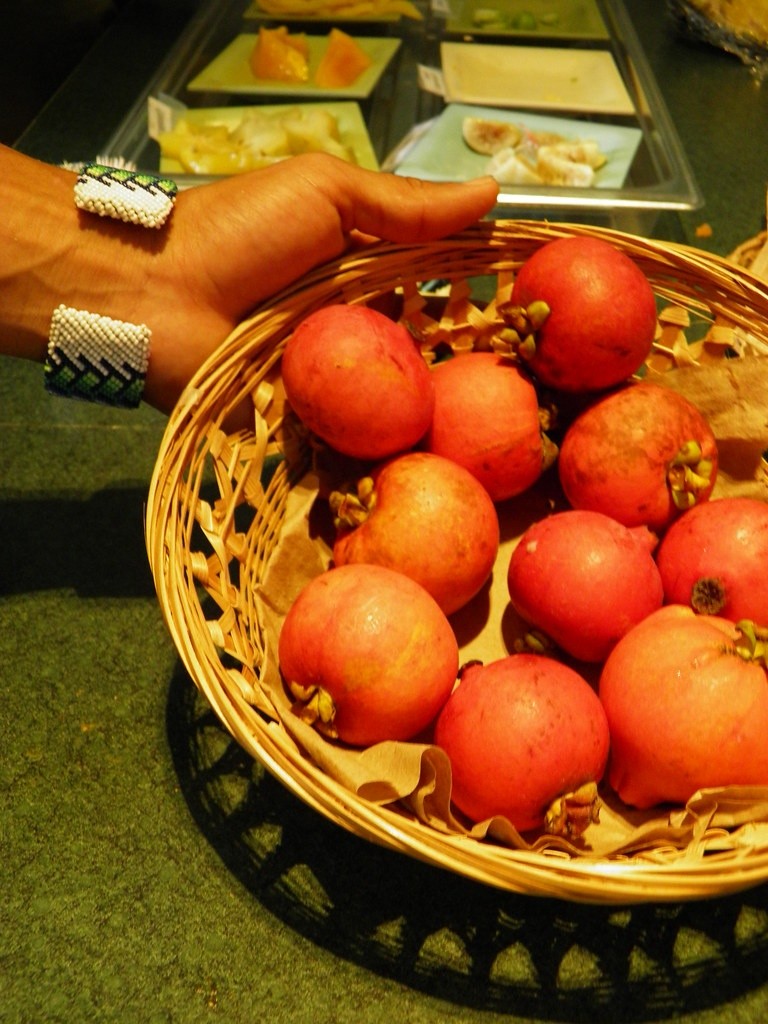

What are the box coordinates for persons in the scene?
[1,143,500,421]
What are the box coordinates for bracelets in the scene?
[44,161,177,412]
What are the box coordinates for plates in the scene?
[240,0,404,22]
[438,41,638,118]
[186,33,405,98]
[391,104,644,190]
[155,100,382,179]
[444,0,611,40]
